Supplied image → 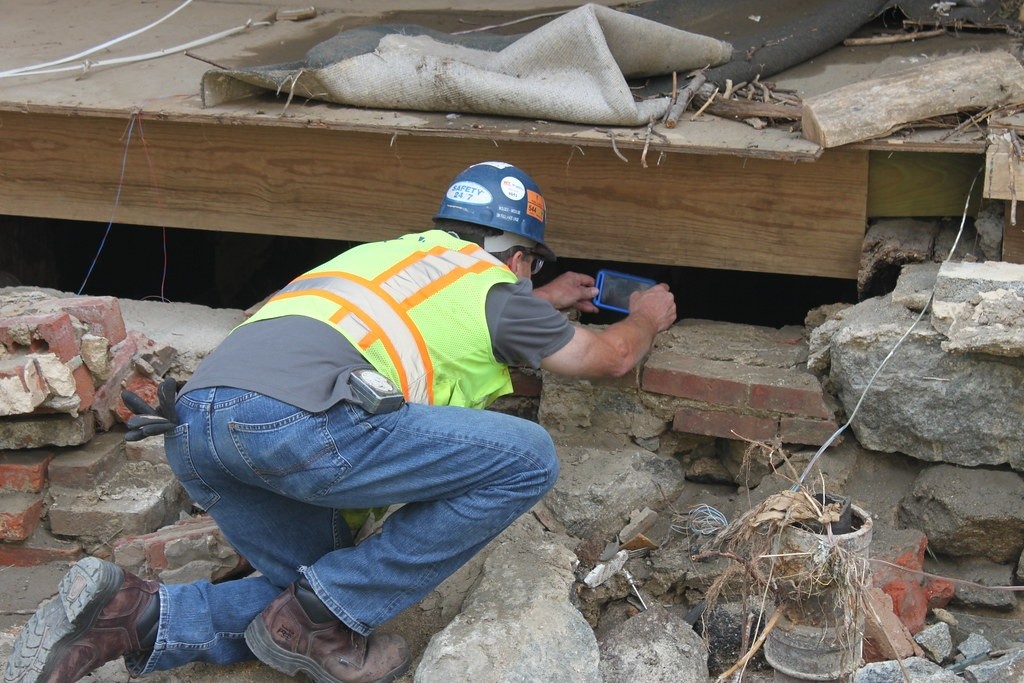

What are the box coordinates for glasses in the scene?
[532,255,544,275]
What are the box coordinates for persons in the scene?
[0,160,677,683]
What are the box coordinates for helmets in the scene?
[432,161,556,261]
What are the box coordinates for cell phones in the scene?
[593,269,656,313]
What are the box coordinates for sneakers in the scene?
[4,557,160,683]
[245,583,412,683]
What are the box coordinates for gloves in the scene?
[121,377,181,443]
